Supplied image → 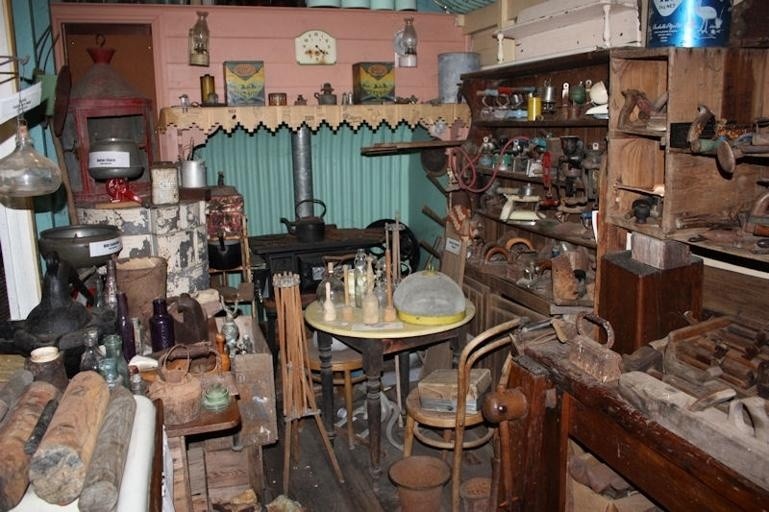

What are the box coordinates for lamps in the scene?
[394,17,418,69]
[188,11,211,67]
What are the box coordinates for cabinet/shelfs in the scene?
[248,225,393,298]
[446,47,769,511]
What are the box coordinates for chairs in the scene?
[304,253,368,451]
[403,315,530,511]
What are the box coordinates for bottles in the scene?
[315,248,387,309]
[0,124,62,198]
[78,259,176,395]
[202,382,232,413]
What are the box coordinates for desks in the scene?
[304,293,475,480]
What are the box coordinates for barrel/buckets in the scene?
[388,455,452,512]
[181,161,207,189]
[437,52,479,104]
[646,0,730,48]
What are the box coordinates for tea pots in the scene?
[280,199,327,243]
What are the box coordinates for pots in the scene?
[208,230,243,271]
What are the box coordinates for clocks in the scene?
[293,29,337,66]
